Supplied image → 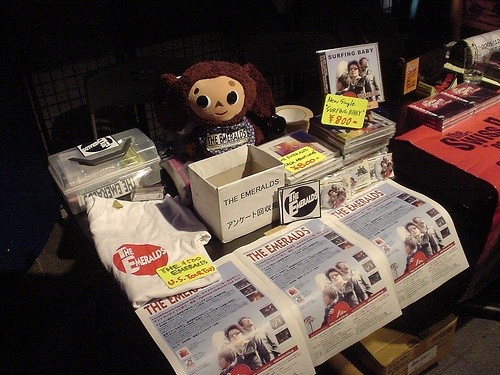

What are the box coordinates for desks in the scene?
[74,172,472,375]
[389,87,500,323]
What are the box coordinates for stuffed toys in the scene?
[159,60,277,161]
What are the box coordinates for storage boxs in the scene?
[45,126,161,217]
[348,302,457,375]
[187,143,285,245]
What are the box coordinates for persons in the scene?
[403,236,426,275]
[405,221,433,259]
[413,216,444,254]
[327,268,360,308]
[360,58,379,100]
[336,261,371,302]
[347,60,372,101]
[328,184,344,207]
[380,156,393,178]
[224,323,266,370]
[238,317,281,364]
[321,284,352,328]
[216,343,252,375]
[336,74,357,99]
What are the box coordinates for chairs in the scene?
[82,54,199,140]
[353,13,412,63]
[245,29,339,118]
